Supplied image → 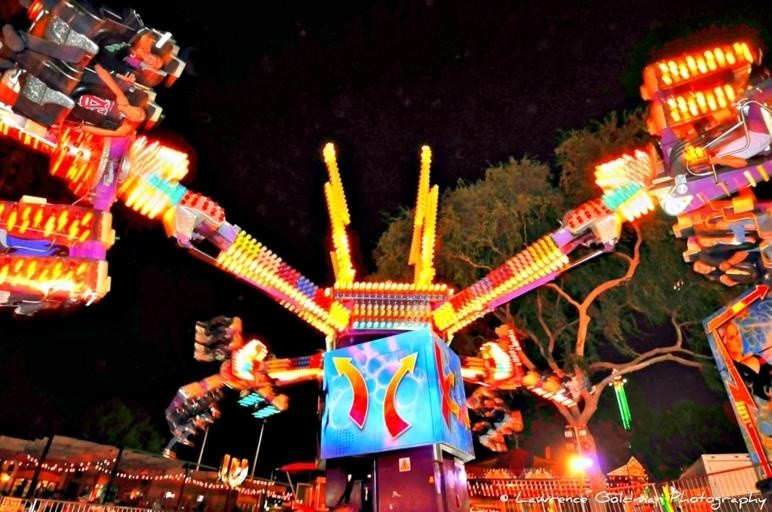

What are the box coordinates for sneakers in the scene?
[3,24,25,52]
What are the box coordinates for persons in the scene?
[0,0,165,139]
[720,320,772,401]
[193,316,233,362]
[558,376,596,394]
[470,408,515,453]
[692,253,757,288]
[63,471,83,501]
[169,391,222,446]
[193,499,207,512]
